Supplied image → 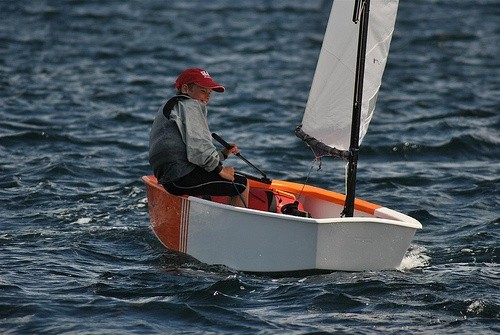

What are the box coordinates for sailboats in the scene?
[142,0,423,272]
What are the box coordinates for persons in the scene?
[149,68,249,209]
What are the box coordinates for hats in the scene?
[175,68,225,92]
